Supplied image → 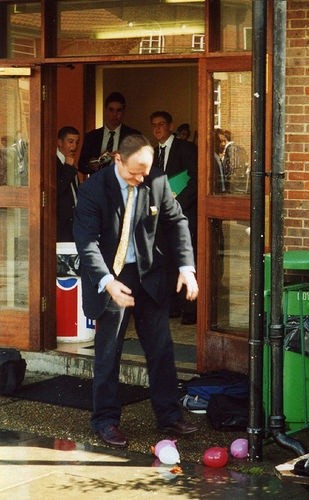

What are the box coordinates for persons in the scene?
[79,134,198,447]
[211,130,248,296]
[57,126,80,277]
[0,134,29,308]
[151,111,198,325]
[80,94,142,175]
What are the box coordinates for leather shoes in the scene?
[159,417,198,435]
[98,425,129,447]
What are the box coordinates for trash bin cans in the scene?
[262,251,309,433]
[56,242,96,343]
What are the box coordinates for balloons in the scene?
[155,439,181,464]
[202,446,229,468]
[230,438,248,458]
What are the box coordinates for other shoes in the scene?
[170,309,181,318]
[182,312,197,325]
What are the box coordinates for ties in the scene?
[220,158,230,191]
[159,145,167,171]
[105,130,116,153]
[71,178,82,199]
[113,185,137,276]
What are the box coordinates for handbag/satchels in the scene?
[0,347,27,395]
[207,393,251,432]
[175,367,250,409]
